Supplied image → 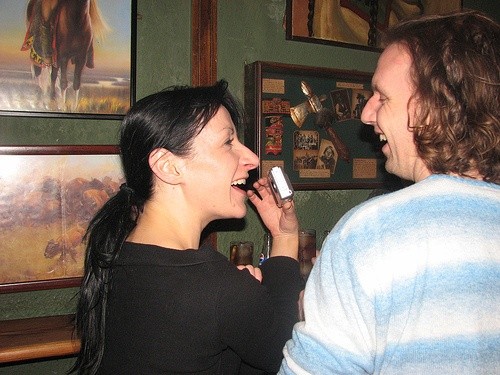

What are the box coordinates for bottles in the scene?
[321,230,330,248]
[258,234,274,268]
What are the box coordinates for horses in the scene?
[25,0,111,112]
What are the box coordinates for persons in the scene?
[276,10,500,374]
[321,146,335,175]
[68,77,304,375]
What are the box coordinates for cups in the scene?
[297,228,318,284]
[229,241,254,267]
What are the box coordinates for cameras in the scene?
[268,167,294,207]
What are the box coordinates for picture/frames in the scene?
[0,0,138,121]
[0,145,128,294]
[285,0,465,53]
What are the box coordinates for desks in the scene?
[0,314,82,364]
